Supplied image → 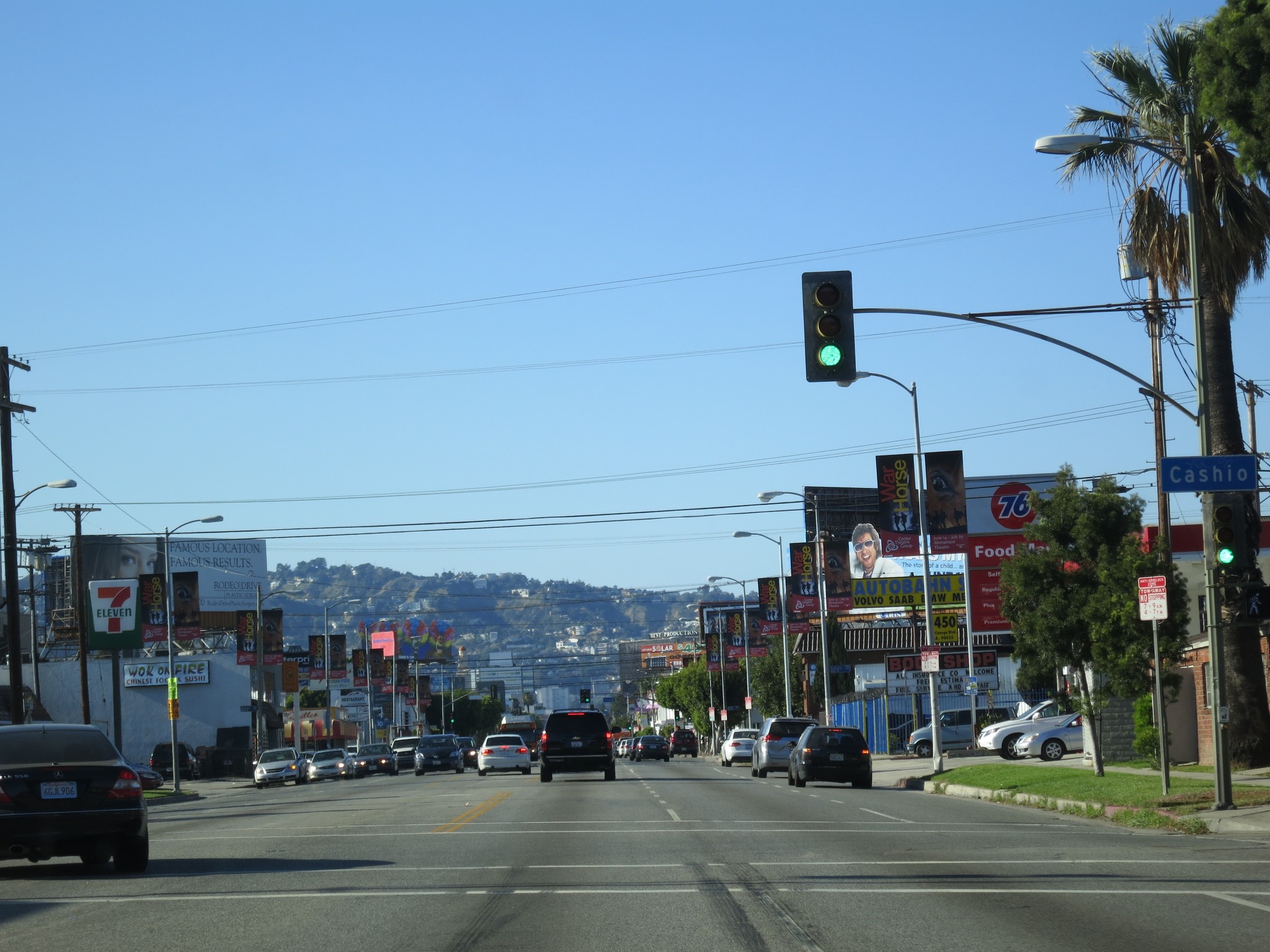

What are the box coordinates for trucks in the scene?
[495,714,540,762]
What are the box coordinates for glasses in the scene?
[854,540,875,552]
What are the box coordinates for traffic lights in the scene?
[628,722,633,732]
[800,268,858,383]
[449,711,457,731]
[1209,502,1243,570]
[580,689,590,703]
[674,710,681,720]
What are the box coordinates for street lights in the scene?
[1035,110,1238,811]
[3,479,77,725]
[392,635,471,742]
[163,515,225,793]
[325,599,362,748]
[838,370,946,774]
[256,583,303,762]
[611,489,834,755]
[365,618,390,743]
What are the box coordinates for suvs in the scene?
[150,742,202,781]
[533,708,622,782]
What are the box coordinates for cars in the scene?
[0,723,149,875]
[719,716,872,789]
[253,733,532,789]
[121,754,164,789]
[613,728,699,761]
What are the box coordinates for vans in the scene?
[908,698,1084,760]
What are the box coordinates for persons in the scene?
[851,523,905,579]
